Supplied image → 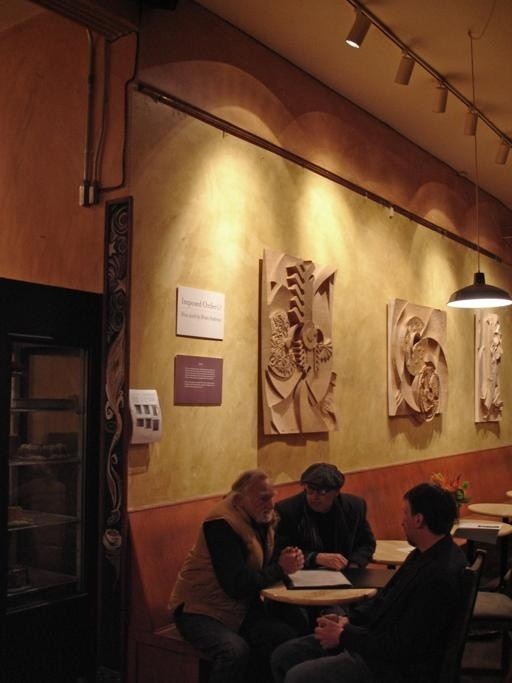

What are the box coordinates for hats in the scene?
[301,463,344,487]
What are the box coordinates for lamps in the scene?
[447,30,512,308]
[346,0,512,165]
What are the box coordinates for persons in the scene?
[268,482,468,681]
[168,470,304,682]
[270,462,376,640]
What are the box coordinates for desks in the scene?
[259,490,512,633]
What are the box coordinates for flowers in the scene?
[431,472,472,508]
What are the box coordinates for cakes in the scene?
[18,442,69,458]
[7,506,24,522]
[7,560,27,588]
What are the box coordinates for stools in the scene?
[460,592,512,677]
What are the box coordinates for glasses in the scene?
[304,488,331,495]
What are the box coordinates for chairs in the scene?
[437,548,487,683]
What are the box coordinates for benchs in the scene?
[127,445,512,683]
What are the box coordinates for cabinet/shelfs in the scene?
[0,278,105,683]
